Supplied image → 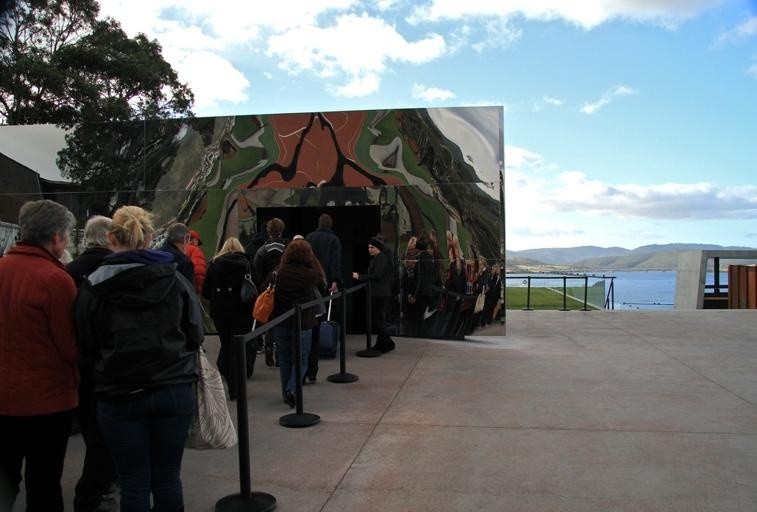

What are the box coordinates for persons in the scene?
[352,233,396,353]
[400,230,504,340]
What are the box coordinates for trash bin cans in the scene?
[728,264,757,309]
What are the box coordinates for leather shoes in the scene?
[284,391,296,409]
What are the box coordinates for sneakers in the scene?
[264,345,274,367]
[92,496,117,512]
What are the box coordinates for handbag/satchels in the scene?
[183,344,238,451]
[240,258,259,306]
[253,270,279,324]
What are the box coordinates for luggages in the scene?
[215,318,257,379]
[317,290,339,359]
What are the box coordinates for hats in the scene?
[188,230,202,246]
